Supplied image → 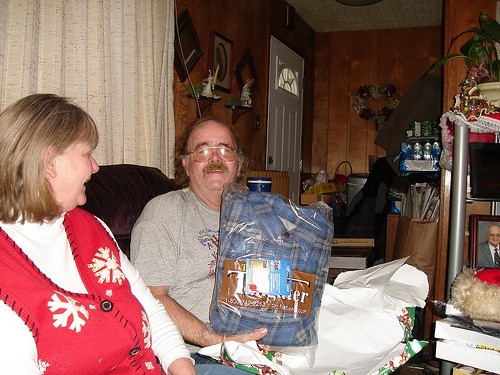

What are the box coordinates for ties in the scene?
[494,248,500,268]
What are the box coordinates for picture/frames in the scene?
[402,133,442,174]
[469,213,500,270]
[208,30,234,93]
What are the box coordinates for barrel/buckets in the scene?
[347,173,369,208]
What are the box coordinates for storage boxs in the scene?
[301,181,336,207]
[434,318,500,374]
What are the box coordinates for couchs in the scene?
[78,163,181,256]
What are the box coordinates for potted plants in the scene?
[427,13,500,107]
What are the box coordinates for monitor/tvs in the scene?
[469,141,500,201]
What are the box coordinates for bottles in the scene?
[315,170,326,183]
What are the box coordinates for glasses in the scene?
[185,146,239,162]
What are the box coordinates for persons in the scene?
[0,92,260,375]
[476,224,500,267]
[127,117,268,364]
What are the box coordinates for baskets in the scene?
[332,160,353,194]
